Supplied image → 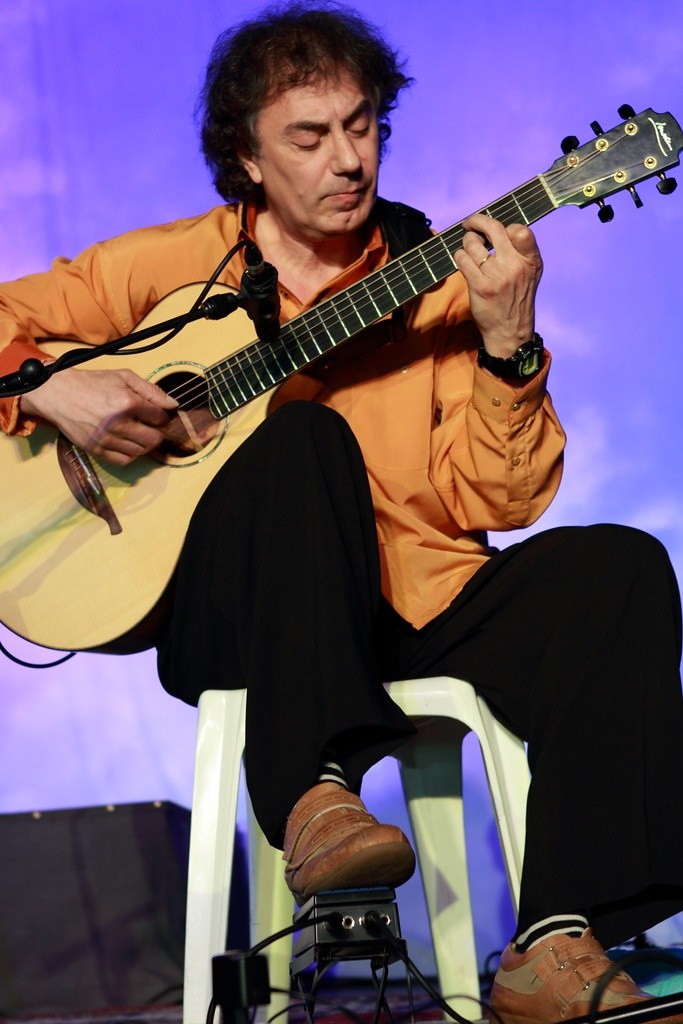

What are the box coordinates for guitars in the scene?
[0,97,683,660]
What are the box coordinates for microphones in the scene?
[243,238,282,344]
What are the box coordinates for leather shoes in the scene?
[490,927,683,1024]
[281,783,417,907]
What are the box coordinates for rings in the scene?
[479,255,491,267]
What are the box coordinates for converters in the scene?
[211,952,270,1008]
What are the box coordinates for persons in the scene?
[0,8,683,1024]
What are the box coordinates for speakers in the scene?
[0,802,248,1024]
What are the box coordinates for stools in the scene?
[184,675,536,1023]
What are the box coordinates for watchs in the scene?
[477,334,544,386]
